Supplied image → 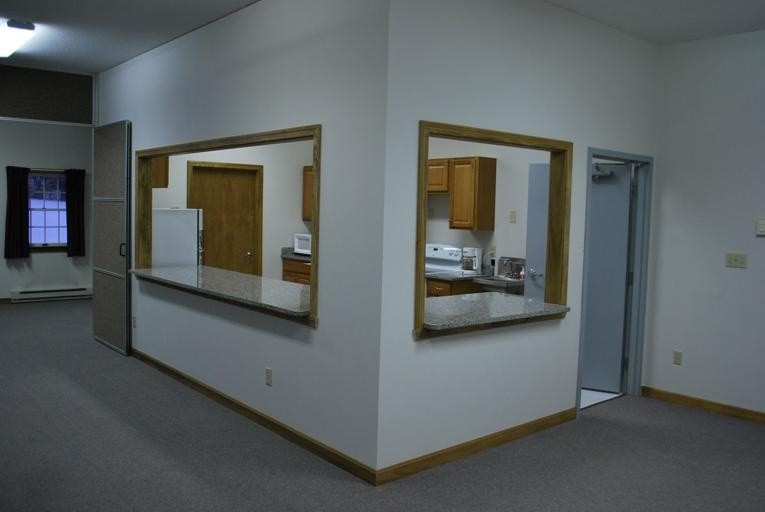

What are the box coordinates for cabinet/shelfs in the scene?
[447,155,497,234]
[425,279,474,296]
[427,158,450,196]
[280,257,311,284]
[150,154,170,189]
[302,164,315,222]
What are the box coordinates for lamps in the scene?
[0,18,37,58]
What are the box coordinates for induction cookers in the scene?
[425,266,455,274]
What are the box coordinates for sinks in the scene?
[473,276,524,294]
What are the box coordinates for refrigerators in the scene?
[151,205,204,267]
[148,266,202,289]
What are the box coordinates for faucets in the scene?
[504,258,513,278]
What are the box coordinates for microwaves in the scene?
[292,232,311,255]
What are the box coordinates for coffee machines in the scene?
[461,246,483,276]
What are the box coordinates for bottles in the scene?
[518,266,525,281]
